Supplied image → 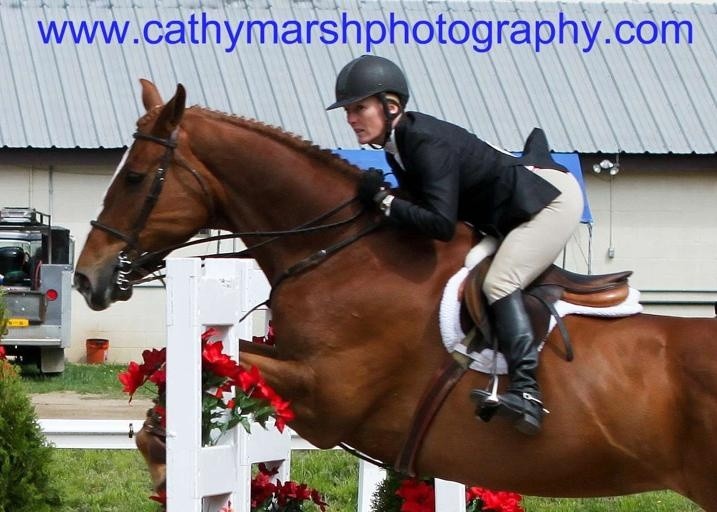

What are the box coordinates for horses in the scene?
[70,75,717,512]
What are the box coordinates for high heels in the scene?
[470,289,545,436]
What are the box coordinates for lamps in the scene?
[592,157,619,176]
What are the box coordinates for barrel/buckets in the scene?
[86,339,109,365]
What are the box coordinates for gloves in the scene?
[355,167,388,209]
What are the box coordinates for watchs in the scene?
[379,195,394,215]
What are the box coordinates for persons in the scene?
[322,54,585,436]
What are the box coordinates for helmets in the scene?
[326,55,410,110]
